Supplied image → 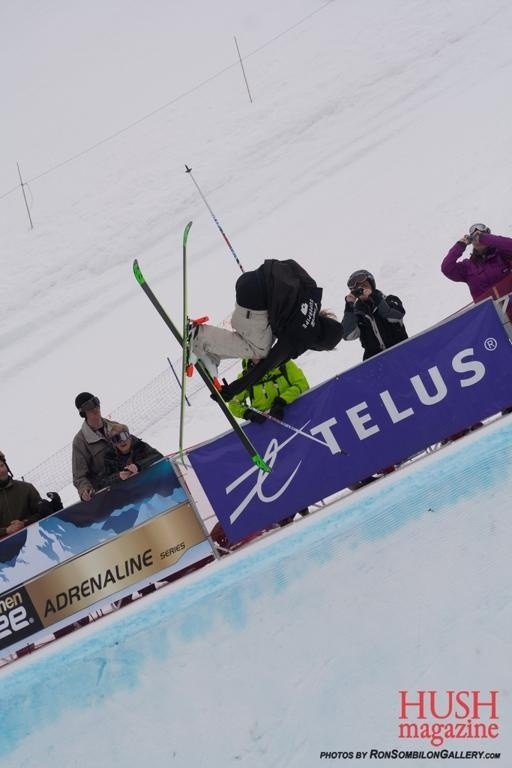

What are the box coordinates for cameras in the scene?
[350,288,364,298]
[467,235,476,243]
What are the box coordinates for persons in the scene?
[341,270,408,361]
[228,355,310,528]
[0,451,74,658]
[186,259,344,402]
[72,392,157,610]
[441,223,512,416]
[96,424,214,582]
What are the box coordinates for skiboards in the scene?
[132,222,273,474]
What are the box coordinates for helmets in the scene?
[349,270,375,292]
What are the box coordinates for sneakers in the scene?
[202,355,218,379]
[189,325,200,366]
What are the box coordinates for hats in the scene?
[76,393,96,416]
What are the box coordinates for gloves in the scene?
[245,409,266,424]
[269,396,286,421]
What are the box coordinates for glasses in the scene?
[78,397,100,412]
[469,223,486,234]
[110,432,129,444]
[347,274,367,288]
